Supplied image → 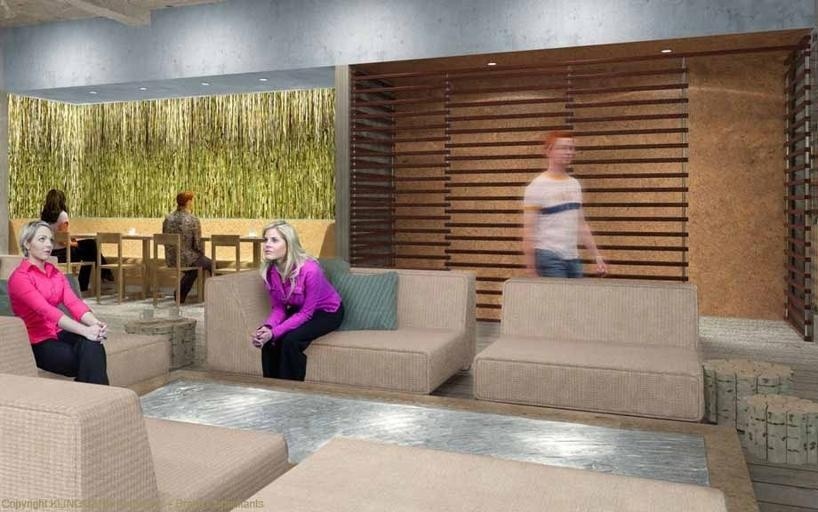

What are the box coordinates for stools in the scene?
[701,357,818,468]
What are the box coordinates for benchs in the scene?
[204,258,707,424]
[227,431,726,511]
[0,315,289,508]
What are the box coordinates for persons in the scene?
[7,221,110,387]
[41,190,115,292]
[162,191,222,303]
[251,218,342,384]
[520,132,607,277]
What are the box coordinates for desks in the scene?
[86,230,265,303]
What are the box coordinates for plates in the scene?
[137,318,160,324]
[165,317,183,321]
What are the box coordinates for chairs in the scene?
[46,233,243,304]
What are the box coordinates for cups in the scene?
[139,308,154,319]
[169,308,183,318]
